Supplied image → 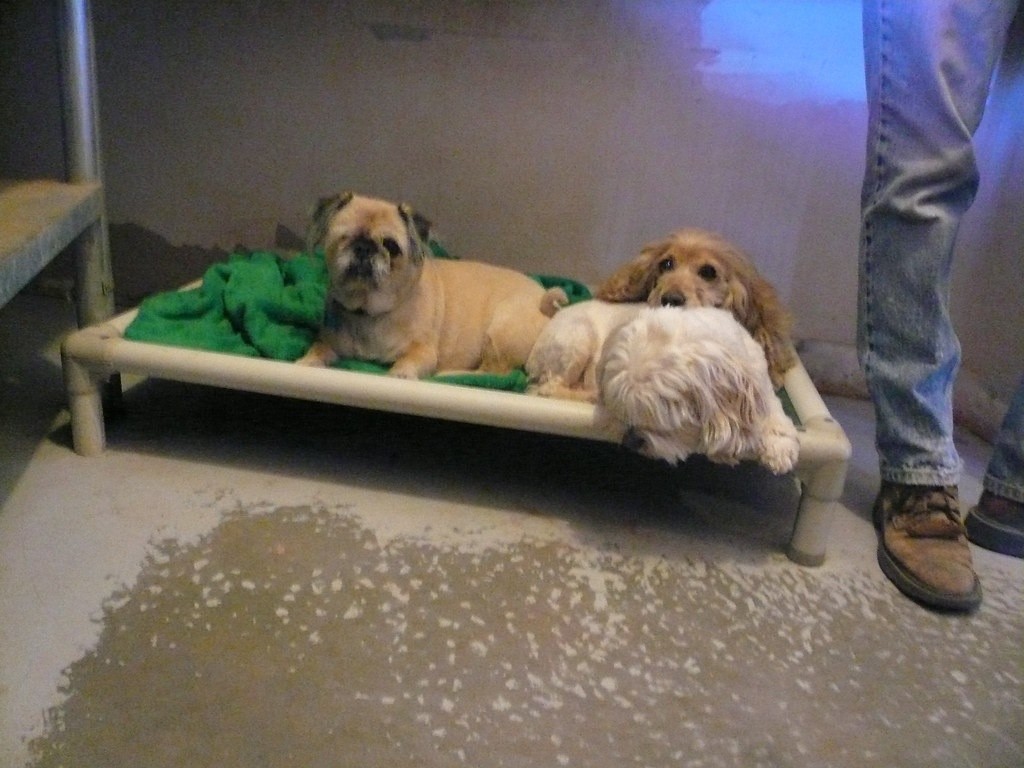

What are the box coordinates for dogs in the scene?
[524,298,800,476]
[523,229,796,401]
[290,191,570,381]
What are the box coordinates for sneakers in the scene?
[871,478,982,613]
[964,488,1024,558]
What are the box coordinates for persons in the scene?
[854,0,1024,610]
